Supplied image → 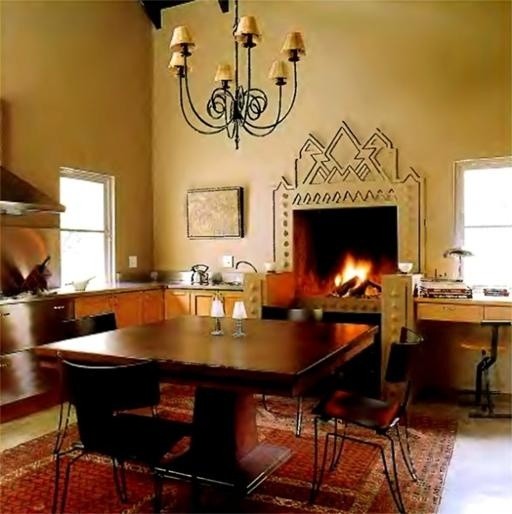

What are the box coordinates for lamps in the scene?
[169,1,308,152]
[208,298,227,335]
[232,299,248,338]
[442,245,477,282]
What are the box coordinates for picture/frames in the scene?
[183,183,245,243]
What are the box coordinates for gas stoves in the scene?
[167,280,242,289]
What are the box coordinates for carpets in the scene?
[0,384,462,514]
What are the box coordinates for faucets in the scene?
[234,260,259,273]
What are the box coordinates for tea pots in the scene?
[190,264,210,282]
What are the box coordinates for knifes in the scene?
[40,255,51,273]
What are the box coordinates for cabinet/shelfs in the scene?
[141,286,164,323]
[413,294,512,420]
[164,286,189,317]
[73,291,142,327]
[190,289,243,319]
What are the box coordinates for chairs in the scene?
[259,303,327,438]
[53,310,117,454]
[306,324,430,513]
[47,349,203,512]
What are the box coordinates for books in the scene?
[484,285,511,296]
[420,280,473,299]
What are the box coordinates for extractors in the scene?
[0,164,67,216]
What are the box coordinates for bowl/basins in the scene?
[73,281,88,291]
[398,263,413,273]
[264,261,279,273]
